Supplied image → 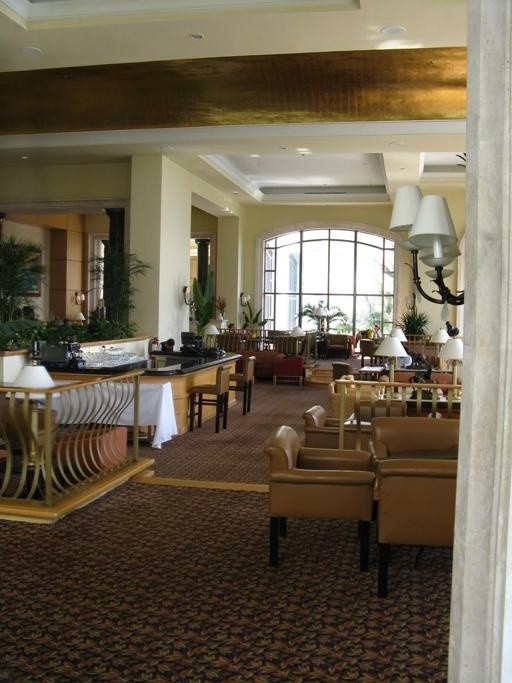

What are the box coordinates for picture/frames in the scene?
[0,245,42,297]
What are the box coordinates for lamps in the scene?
[74,291,85,304]
[386,184,465,306]
[75,311,86,326]
[4,359,62,466]
[315,307,327,335]
[205,323,220,348]
[241,291,252,306]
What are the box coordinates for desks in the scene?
[42,379,172,443]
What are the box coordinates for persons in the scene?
[409,375,440,400]
[374,375,390,399]
[227,323,383,350]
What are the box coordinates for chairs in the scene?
[368,416,459,592]
[216,323,326,385]
[188,363,232,432]
[302,404,354,448]
[266,424,374,572]
[326,324,462,418]
[229,357,257,415]
